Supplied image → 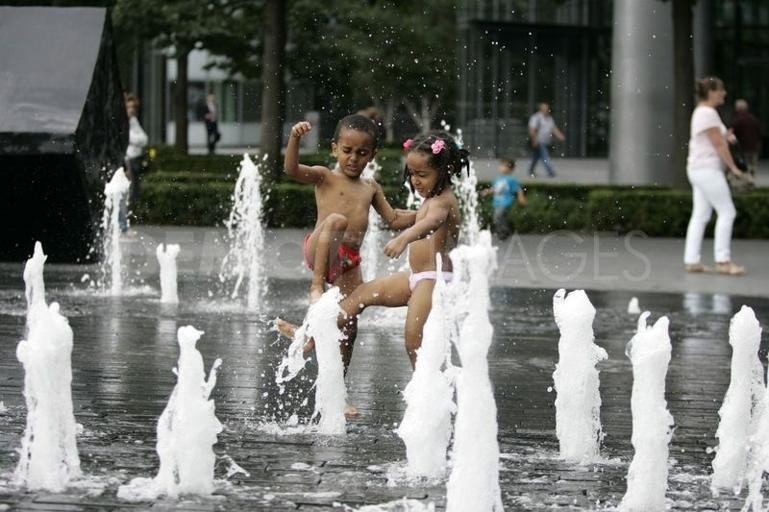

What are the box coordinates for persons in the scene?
[480,157,527,241]
[284,114,418,417]
[118,91,149,238]
[527,102,566,177]
[198,87,220,153]
[684,75,748,275]
[727,98,762,185]
[271,130,471,372]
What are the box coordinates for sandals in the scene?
[716,261,748,276]
[686,263,715,272]
